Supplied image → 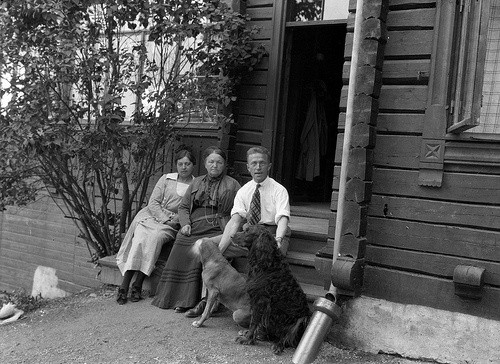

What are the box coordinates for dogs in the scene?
[230,225,314,355]
[192,237,252,329]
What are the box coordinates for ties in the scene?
[249,184,262,225]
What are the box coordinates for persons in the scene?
[150,145,242,312]
[116,150,196,305]
[185,146,291,326]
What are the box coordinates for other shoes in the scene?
[115,286,129,304]
[174,307,183,313]
[131,282,142,302]
[254,330,267,340]
[185,300,215,318]
